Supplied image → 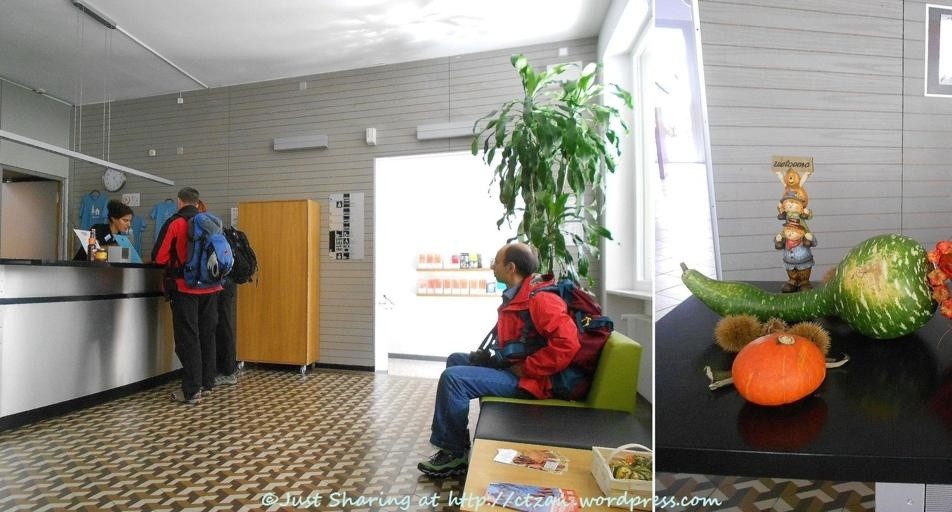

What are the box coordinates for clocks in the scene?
[100,163,127,194]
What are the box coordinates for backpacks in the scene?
[223,227,257,283]
[177,211,234,289]
[519,280,614,400]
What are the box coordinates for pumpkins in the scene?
[730,333,828,406]
[609,454,651,481]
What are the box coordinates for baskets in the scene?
[590,443,652,511]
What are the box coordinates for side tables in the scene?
[453,385,655,511]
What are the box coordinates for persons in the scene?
[151,187,238,405]
[73,200,133,260]
[418,243,581,479]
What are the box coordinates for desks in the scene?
[655,280,952,512]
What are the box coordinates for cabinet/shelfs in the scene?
[235,196,320,375]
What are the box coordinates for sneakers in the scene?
[418,448,468,477]
[171,368,240,404]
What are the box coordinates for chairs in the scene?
[480,330,644,413]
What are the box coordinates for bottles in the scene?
[87,229,96,262]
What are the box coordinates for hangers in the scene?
[89,182,100,196]
